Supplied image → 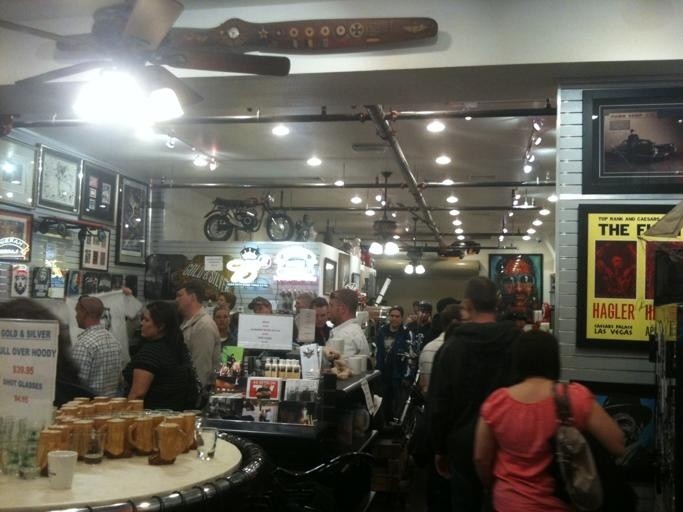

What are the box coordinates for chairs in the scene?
[368,172,400,255]
[253,366,426,512]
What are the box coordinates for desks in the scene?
[0,428,265,511]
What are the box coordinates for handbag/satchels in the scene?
[555,426,639,511]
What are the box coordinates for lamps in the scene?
[404,207,425,275]
[70,52,183,136]
[496,119,558,244]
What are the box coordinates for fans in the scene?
[0,0,290,150]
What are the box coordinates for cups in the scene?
[0,395,220,490]
[344,357,361,376]
[525,310,553,334]
[328,339,344,355]
[354,354,370,371]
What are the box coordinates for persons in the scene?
[627,128,640,165]
[70,277,626,511]
[495,255,539,320]
[14,270,28,296]
[33,268,49,293]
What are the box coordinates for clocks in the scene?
[32,143,83,216]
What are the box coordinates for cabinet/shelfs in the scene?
[200,352,326,441]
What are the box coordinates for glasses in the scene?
[504,274,535,291]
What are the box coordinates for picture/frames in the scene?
[0,134,35,215]
[322,252,361,296]
[0,211,32,263]
[578,204,682,350]
[489,254,543,312]
[582,90,683,194]
[81,160,149,272]
[567,379,677,487]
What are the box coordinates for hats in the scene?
[249,296,271,309]
[437,298,462,313]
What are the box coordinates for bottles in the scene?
[264,358,300,380]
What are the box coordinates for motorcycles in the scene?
[202,190,295,241]
[232,369,430,510]
